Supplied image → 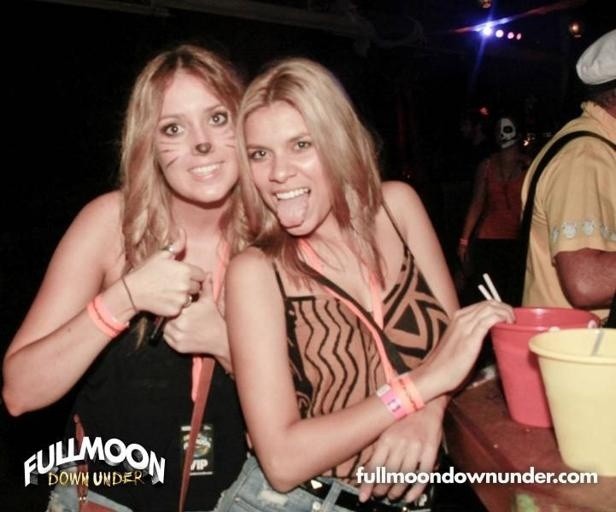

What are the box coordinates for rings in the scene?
[182,296,193,308]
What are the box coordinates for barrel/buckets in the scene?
[527,329,616,476]
[488,306,604,427]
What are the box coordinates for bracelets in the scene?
[458,237,468,246]
[372,382,407,421]
[391,371,426,415]
[83,296,131,341]
[118,272,145,319]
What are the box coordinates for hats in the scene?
[576,28,616,86]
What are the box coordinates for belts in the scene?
[296,477,416,512]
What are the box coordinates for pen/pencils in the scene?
[149,316,165,341]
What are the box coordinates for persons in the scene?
[209,54,520,512]
[509,28,615,329]
[451,106,539,316]
[0,41,249,512]
